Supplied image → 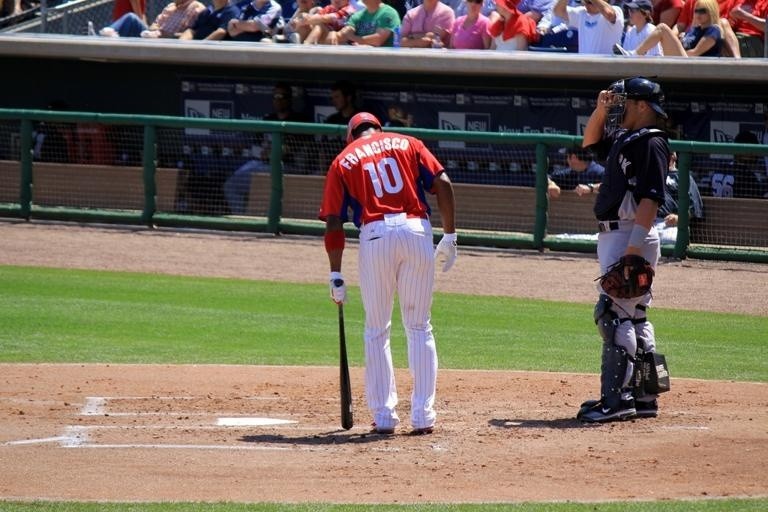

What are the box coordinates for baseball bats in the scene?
[334,279,354,430]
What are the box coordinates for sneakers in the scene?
[576,397,638,423]
[612,43,631,55]
[412,425,434,434]
[581,393,659,419]
[371,422,397,435]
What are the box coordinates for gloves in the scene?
[434,232,458,272]
[329,270,348,307]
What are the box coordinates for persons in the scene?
[321,111,458,434]
[577,75,669,424]
[651,149,705,247]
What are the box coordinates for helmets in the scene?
[616,76,670,119]
[346,111,383,144]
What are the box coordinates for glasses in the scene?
[695,8,708,14]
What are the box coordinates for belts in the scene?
[597,220,619,234]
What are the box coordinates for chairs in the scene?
[529,27,576,52]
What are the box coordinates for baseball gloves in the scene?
[600,254,655,300]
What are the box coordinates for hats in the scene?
[623,0,655,12]
[644,100,668,119]
